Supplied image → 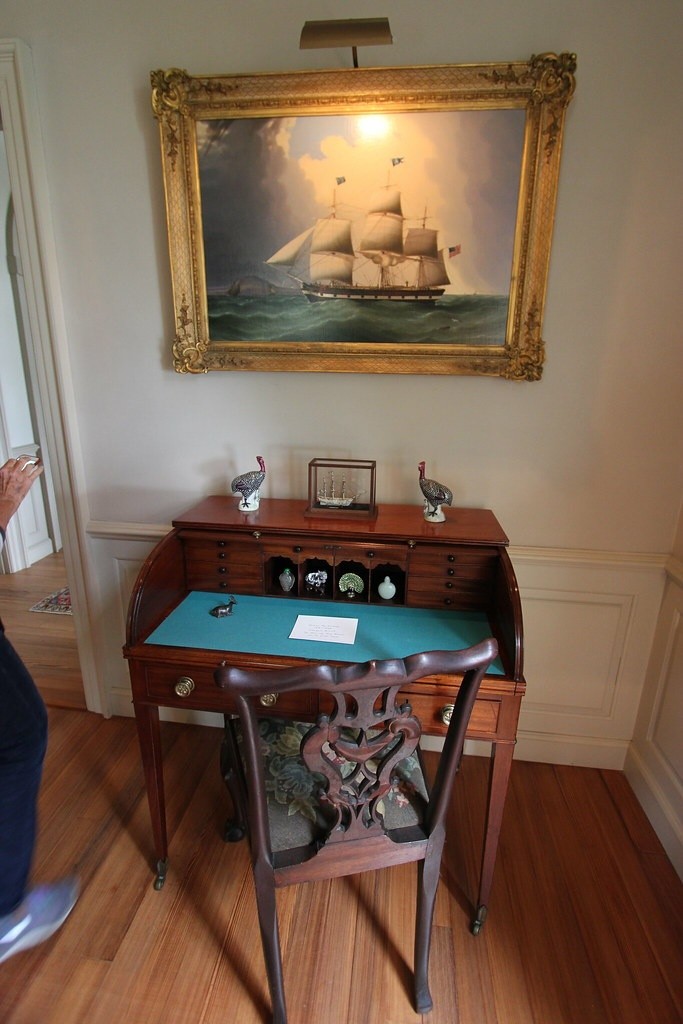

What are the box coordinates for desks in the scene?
[111,496,529,933]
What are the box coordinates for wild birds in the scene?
[230,456,266,508]
[417,461,453,517]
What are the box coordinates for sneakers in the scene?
[0,875,81,963]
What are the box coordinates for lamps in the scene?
[298,17,393,68]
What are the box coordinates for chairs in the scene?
[209,637,500,1024]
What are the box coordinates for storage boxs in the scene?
[303,458,379,520]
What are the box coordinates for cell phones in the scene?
[15,454,40,471]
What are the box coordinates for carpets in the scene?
[27,583,73,615]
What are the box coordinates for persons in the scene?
[0,455,82,964]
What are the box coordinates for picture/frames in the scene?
[145,53,578,384]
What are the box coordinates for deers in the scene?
[210,595,238,618]
